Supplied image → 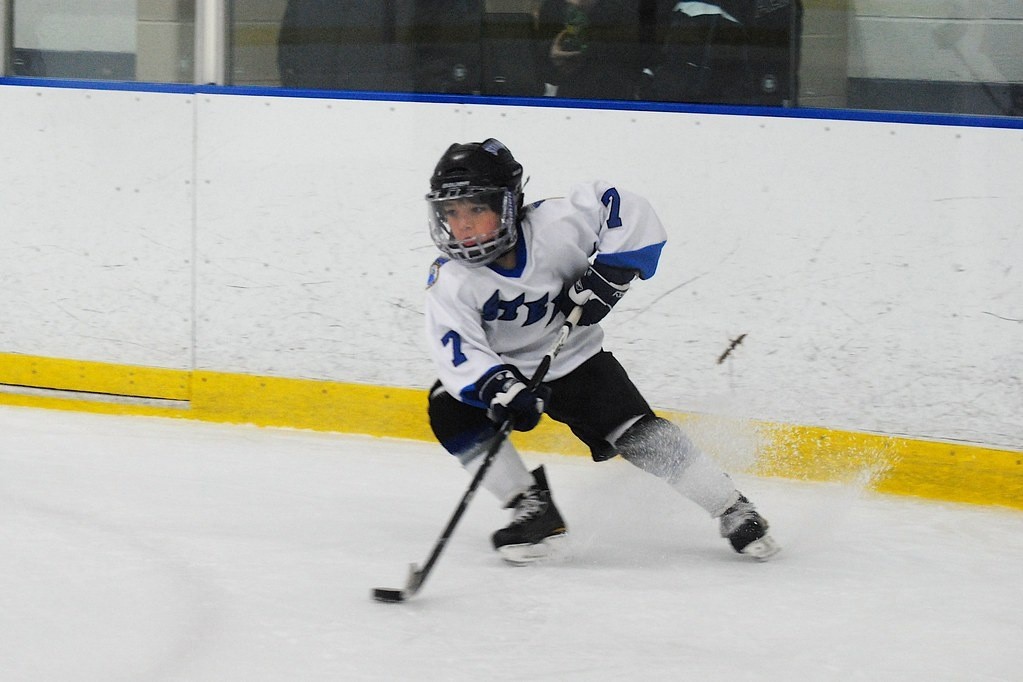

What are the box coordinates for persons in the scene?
[280,0,786,110]
[424,139,784,560]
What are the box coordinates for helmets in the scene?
[424,137,525,267]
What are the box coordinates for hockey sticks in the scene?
[404,305,584,595]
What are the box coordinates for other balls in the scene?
[371,587,406,603]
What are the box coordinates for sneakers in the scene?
[493,465,567,565]
[718,473,783,560]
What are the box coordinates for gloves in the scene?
[558,259,637,326]
[478,362,551,432]
